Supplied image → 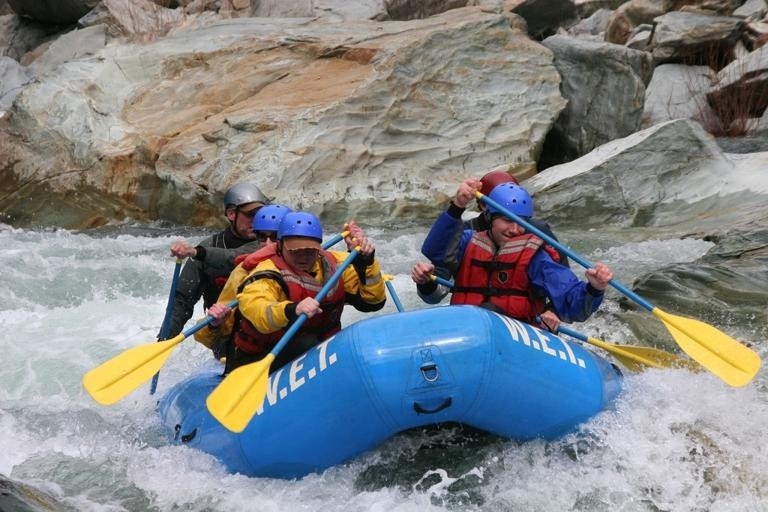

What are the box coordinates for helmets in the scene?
[276,211,323,244]
[250,203,294,232]
[476,169,520,202]
[224,183,266,218]
[485,183,533,218]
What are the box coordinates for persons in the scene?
[230,212,386,377]
[411,171,571,335]
[157,184,271,361]
[206,204,363,377]
[422,179,613,334]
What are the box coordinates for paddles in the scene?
[83,223,354,406]
[429,274,704,376]
[473,190,761,387]
[206,236,362,434]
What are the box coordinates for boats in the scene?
[158,303,622,480]
